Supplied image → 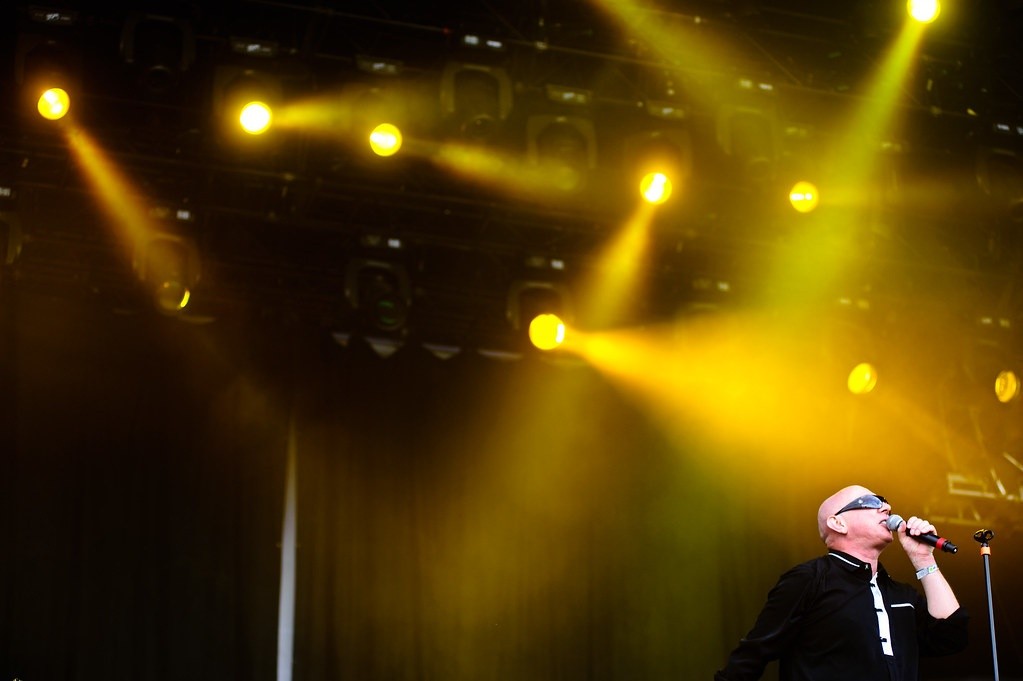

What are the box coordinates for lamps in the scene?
[22,41,72,122]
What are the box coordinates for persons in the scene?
[716,485,968,681]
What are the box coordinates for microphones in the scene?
[886,514,958,554]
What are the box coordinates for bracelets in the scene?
[916,563,939,580]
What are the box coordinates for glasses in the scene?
[833,495,891,516]
